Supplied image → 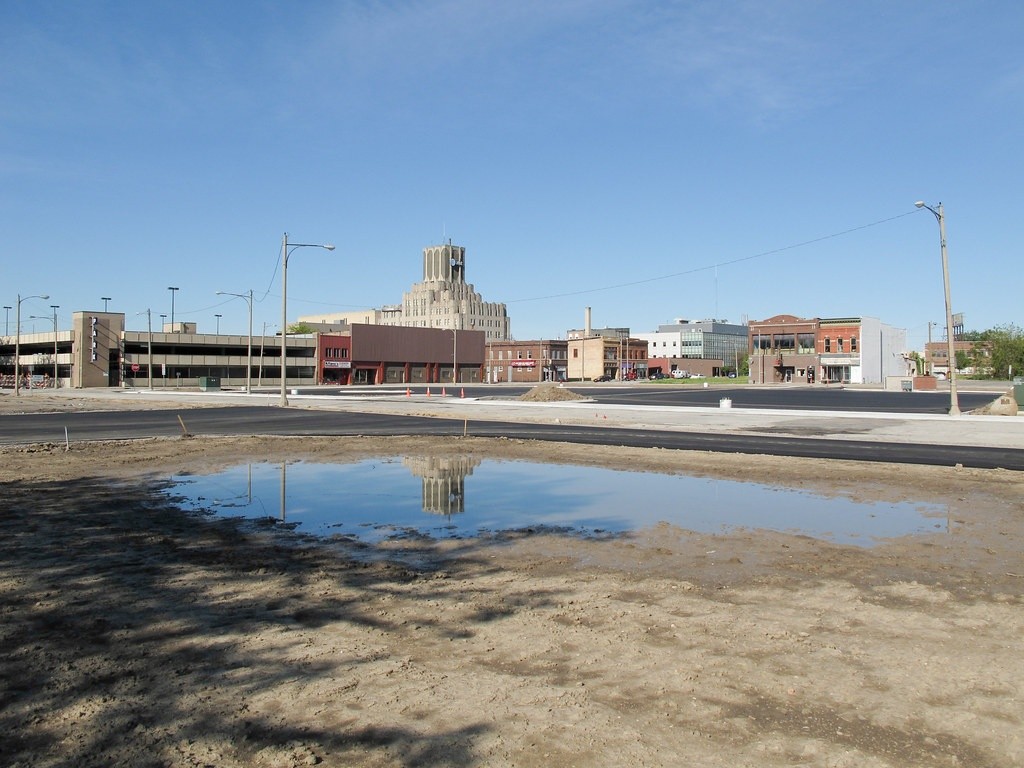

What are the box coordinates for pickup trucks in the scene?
[690,374,706,379]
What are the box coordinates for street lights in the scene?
[915,201,961,415]
[929,321,937,376]
[136,309,153,390]
[280,232,335,407]
[101,297,111,312]
[626,337,628,374]
[489,339,492,384]
[28,313,58,389]
[723,340,738,377]
[50,305,59,332]
[15,294,49,396]
[215,289,253,394]
[160,315,167,332]
[168,287,179,333]
[442,324,456,383]
[258,322,277,386]
[215,315,222,334]
[3,307,12,336]
[220,463,252,507]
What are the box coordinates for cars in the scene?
[728,372,736,378]
[648,373,669,380]
[675,370,689,379]
[593,375,612,382]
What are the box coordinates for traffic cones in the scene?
[426,387,431,397]
[405,387,411,397]
[441,386,446,397]
[460,388,466,398]
